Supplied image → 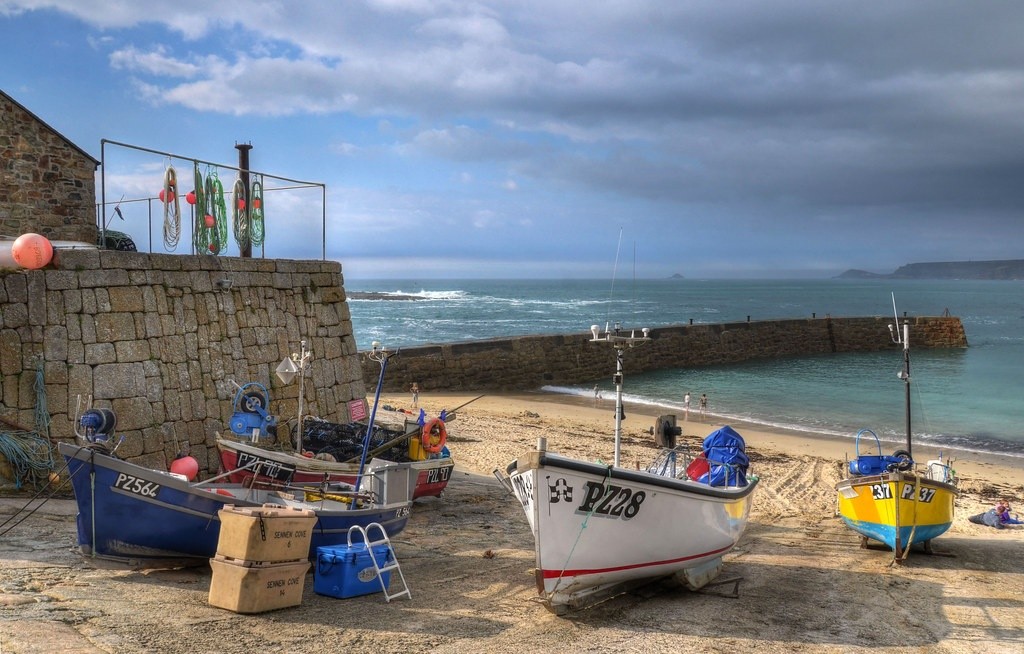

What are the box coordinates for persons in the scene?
[699,394,707,417]
[594,384,599,398]
[999,499,1024,524]
[410,382,420,408]
[684,393,690,411]
[968,506,1011,529]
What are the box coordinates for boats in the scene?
[835,292,959,561]
[492,227,760,616]
[60,342,419,566]
[214,340,454,502]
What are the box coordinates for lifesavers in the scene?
[420,417,448,454]
[891,449,914,472]
